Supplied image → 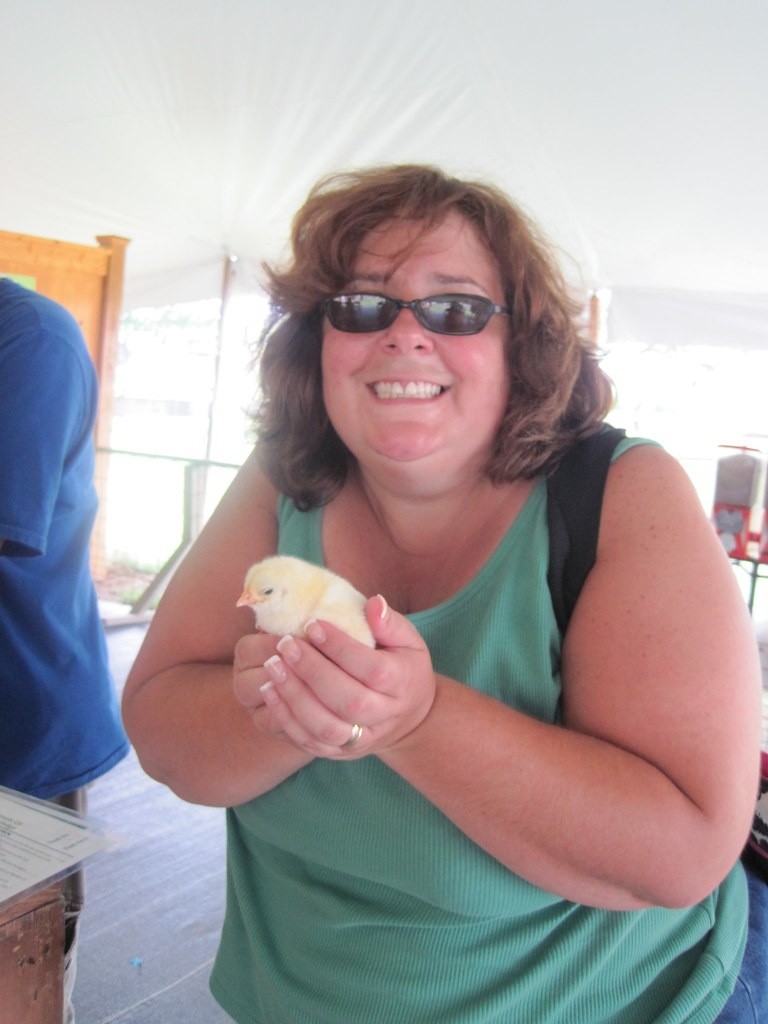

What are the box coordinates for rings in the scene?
[341,725,362,748]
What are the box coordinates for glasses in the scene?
[317,291,512,336]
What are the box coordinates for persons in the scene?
[0,277,130,1024]
[121,161,768,1024]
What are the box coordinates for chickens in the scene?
[235,554,377,654]
